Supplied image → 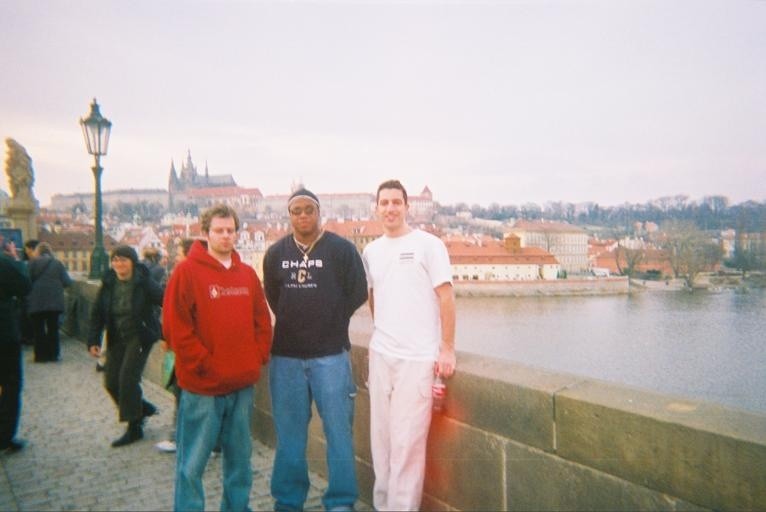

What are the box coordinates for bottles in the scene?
[431,373,448,416]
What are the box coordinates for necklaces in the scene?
[289,227,325,266]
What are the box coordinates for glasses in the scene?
[287,204,318,216]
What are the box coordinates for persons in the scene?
[359,179,460,512]
[82,246,166,446]
[163,203,274,511]
[151,238,225,459]
[0,235,32,457]
[257,183,366,511]
[7,237,168,372]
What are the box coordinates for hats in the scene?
[287,189,320,209]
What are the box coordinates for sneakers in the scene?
[109,396,180,453]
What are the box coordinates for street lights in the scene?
[79,96,113,279]
